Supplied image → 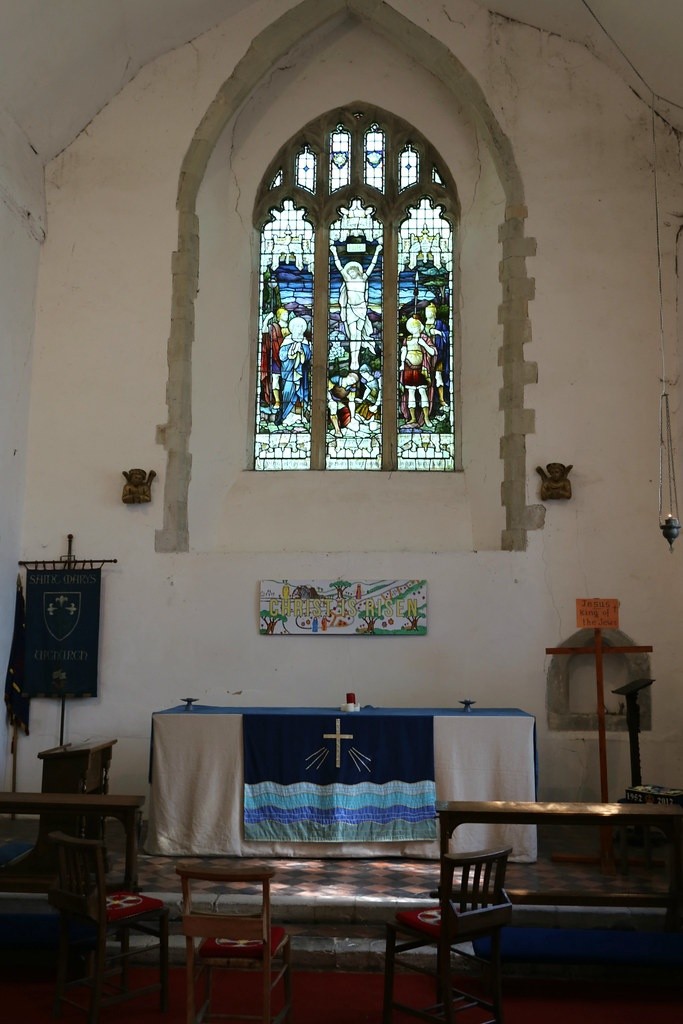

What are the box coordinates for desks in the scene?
[1,790,145,893]
[147,704,539,863]
[433,801,683,931]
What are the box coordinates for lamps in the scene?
[650,90,680,551]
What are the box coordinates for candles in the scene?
[347,693,355,704]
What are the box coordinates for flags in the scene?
[5,585,29,737]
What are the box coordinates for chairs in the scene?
[48,830,168,1024]
[384,846,513,1024]
[175,863,292,1024]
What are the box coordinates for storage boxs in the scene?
[627,785,683,805]
